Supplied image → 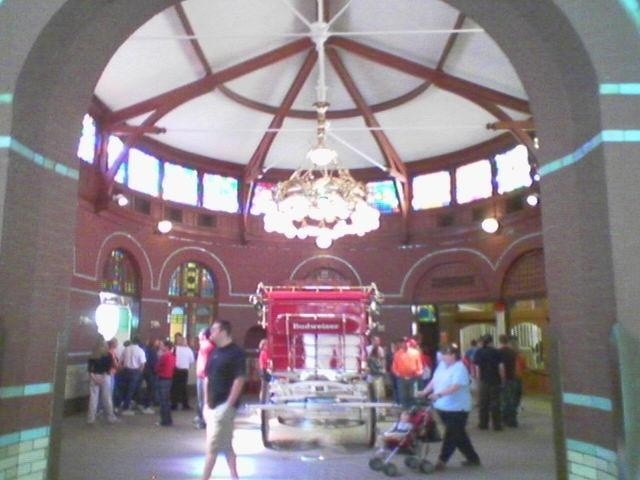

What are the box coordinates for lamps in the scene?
[248,97,382,250]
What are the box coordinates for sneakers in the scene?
[462,460,479,466]
[432,462,444,470]
[86,400,159,423]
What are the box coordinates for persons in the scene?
[452,334,527,431]
[85,330,199,425]
[414,345,480,471]
[366,334,432,409]
[200,318,249,479]
[257,329,272,403]
[193,327,215,428]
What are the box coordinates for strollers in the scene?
[371,403,435,479]
